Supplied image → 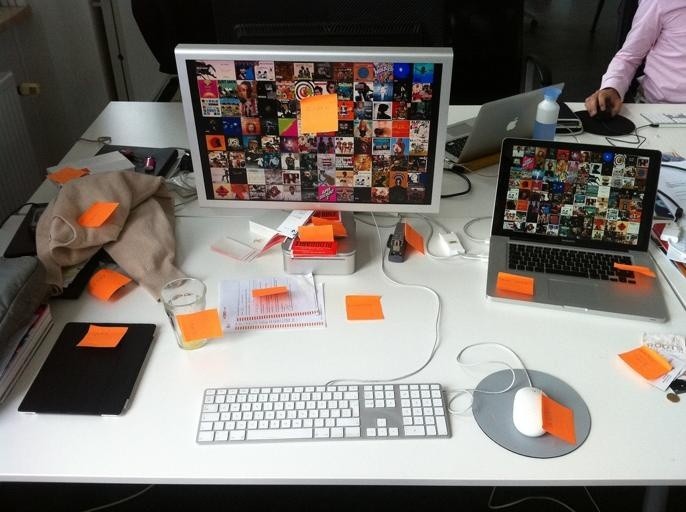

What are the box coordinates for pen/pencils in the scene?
[652,123,686,128]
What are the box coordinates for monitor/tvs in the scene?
[175,44,454,274]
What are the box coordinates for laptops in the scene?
[486,137,669,323]
[445,82,566,164]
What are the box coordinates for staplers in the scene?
[388,222,407,263]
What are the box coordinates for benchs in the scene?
[128,1,556,105]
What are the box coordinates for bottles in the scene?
[532,88,562,140]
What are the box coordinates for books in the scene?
[1,269,157,416]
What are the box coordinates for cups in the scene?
[162,278,209,350]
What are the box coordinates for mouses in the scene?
[595,99,612,122]
[513,387,547,437]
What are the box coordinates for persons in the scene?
[503,147,650,247]
[195,61,432,208]
[584,1,686,125]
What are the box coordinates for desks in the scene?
[2,101,686,487]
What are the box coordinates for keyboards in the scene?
[196,383,451,445]
